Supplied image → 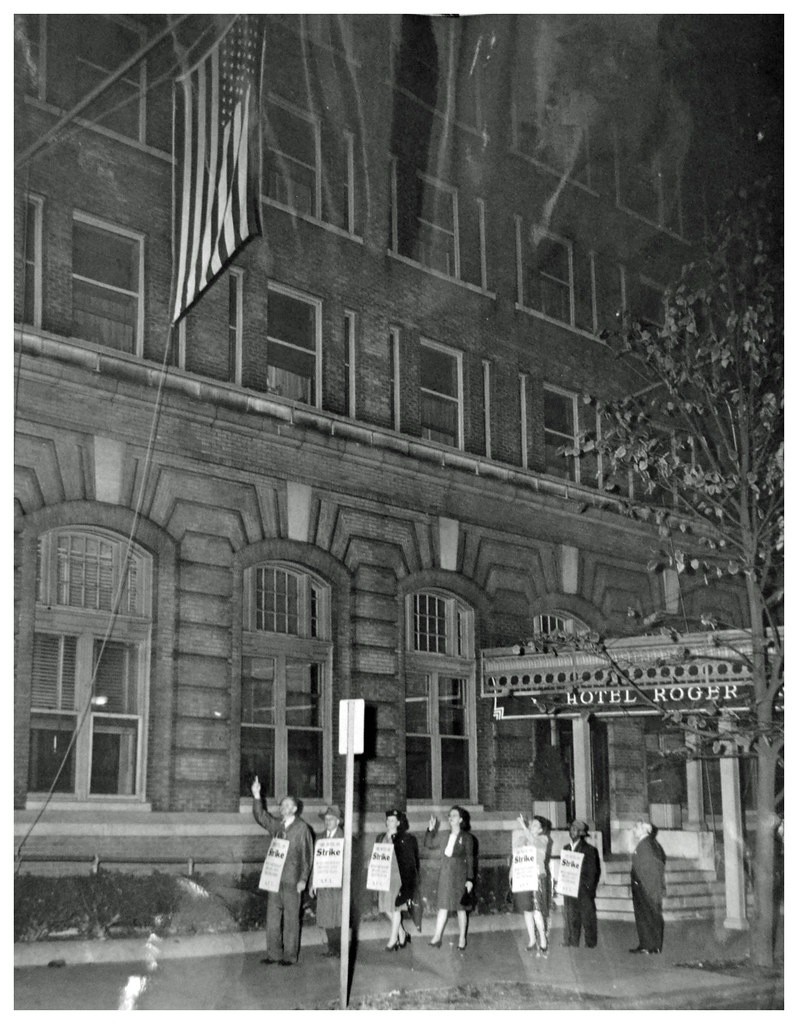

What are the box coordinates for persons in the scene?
[507,812,553,952]
[372,808,421,951]
[629,820,666,953]
[251,776,315,965]
[314,805,358,959]
[423,805,476,951]
[558,820,601,949]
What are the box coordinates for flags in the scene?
[165,14,268,324]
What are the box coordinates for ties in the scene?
[328,832,332,838]
[281,819,285,830]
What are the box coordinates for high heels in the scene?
[427,940,442,949]
[457,939,467,951]
[399,932,411,949]
[385,938,399,951]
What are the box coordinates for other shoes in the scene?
[629,946,649,954]
[525,943,536,951]
[560,942,578,947]
[540,938,548,952]
[261,959,280,964]
[278,960,297,965]
[320,949,339,958]
[584,944,598,950]
[649,948,661,954]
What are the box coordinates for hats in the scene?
[386,809,402,819]
[317,805,343,826]
[566,819,587,835]
